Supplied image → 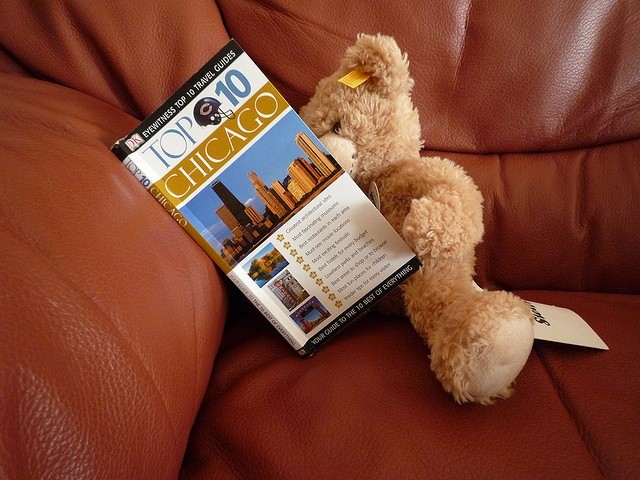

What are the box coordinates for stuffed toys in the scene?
[298,30,536,407]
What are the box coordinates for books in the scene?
[111,37,424,360]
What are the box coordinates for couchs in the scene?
[0,0,640,480]
